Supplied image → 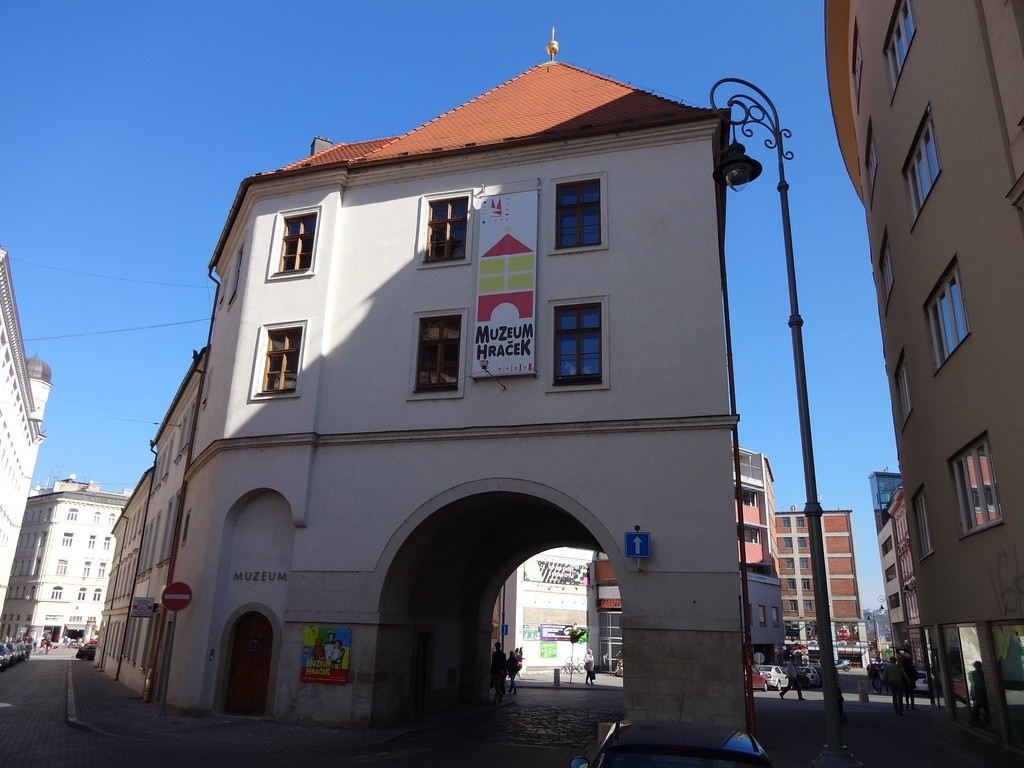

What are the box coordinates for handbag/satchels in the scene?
[590,672,595,680]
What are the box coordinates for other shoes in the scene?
[591,683,593,684]
[911,705,915,710]
[906,704,909,709]
[586,682,589,684]
[798,697,806,701]
[499,695,503,701]
[893,705,898,710]
[779,693,784,699]
[898,711,902,716]
[503,687,506,694]
[494,700,497,704]
[508,690,511,693]
[513,691,517,695]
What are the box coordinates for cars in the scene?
[0,642,30,672]
[755,665,794,691]
[914,671,937,698]
[745,666,768,692]
[834,659,851,672]
[797,659,823,688]
[782,666,811,690]
[867,664,880,678]
[69,638,99,661]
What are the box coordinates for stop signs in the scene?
[162,581,193,613]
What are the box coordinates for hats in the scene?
[888,657,897,664]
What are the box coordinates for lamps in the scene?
[294,516,308,529]
[28,408,43,422]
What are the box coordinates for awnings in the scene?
[66,624,86,630]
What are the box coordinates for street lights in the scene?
[866,609,878,648]
[707,76,861,768]
[877,595,895,658]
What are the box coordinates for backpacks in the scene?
[584,661,592,670]
[509,659,519,673]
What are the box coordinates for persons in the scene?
[506,651,518,694]
[514,647,524,677]
[898,654,918,710]
[869,665,880,693]
[491,642,507,704]
[779,655,806,700]
[616,660,623,674]
[879,669,889,694]
[24,635,29,644]
[883,657,910,716]
[42,630,51,653]
[970,661,992,728]
[585,649,594,684]
